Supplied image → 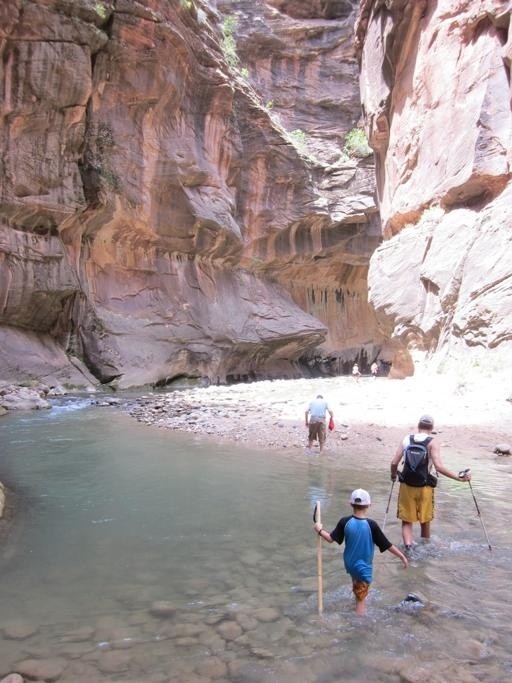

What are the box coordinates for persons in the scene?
[314,488,409,615]
[305,393,335,455]
[352,362,362,383]
[390,415,472,556]
[370,361,378,380]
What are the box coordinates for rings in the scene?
[467,473,468,475]
[466,475,468,478]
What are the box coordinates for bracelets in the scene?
[390,474,396,479]
[318,527,324,536]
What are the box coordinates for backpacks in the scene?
[397,435,437,490]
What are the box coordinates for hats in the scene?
[350,489,371,507]
[419,414,433,429]
